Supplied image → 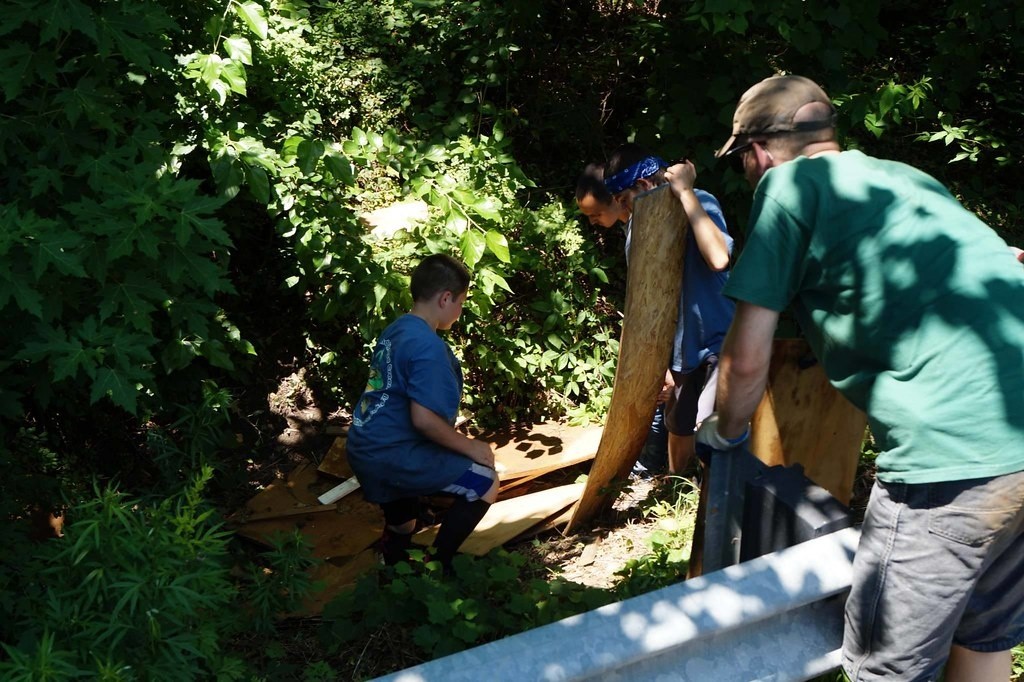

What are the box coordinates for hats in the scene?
[713,74,838,158]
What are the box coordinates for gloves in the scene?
[695,411,750,468]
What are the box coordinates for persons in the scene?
[603,149,734,486]
[345,253,500,566]
[694,71,1024,682]
[576,160,632,270]
